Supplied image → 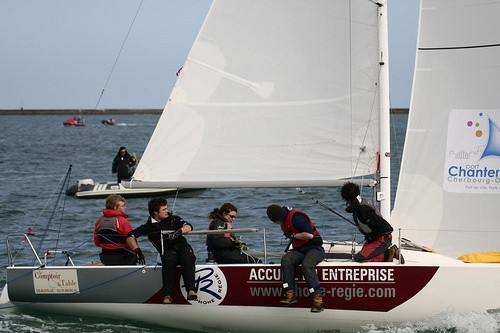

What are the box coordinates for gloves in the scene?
[134,248,145,265]
[166,228,182,240]
[284,231,291,240]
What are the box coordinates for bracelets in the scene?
[292,232,296,239]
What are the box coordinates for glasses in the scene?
[227,213,235,218]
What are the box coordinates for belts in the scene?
[102,247,128,255]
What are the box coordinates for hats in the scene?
[266,205,281,221]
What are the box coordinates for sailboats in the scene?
[5,0,500,333]
[286,0,500,263]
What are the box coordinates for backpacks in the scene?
[97,216,118,234]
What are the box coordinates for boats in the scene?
[63,122,85,127]
[75,179,199,198]
[102,119,115,126]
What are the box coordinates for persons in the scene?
[266,204,326,313]
[93,195,145,266]
[125,198,198,303]
[111,146,138,183]
[206,203,263,264]
[340,181,405,264]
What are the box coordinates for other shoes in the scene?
[279,289,298,304]
[311,293,324,312]
[387,244,405,263]
[187,289,198,300]
[162,295,173,304]
